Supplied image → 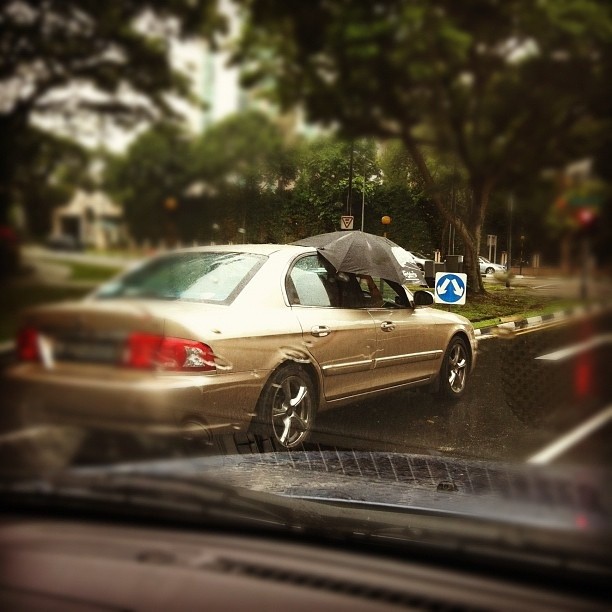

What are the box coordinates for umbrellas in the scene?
[284,226,429,290]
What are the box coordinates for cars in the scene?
[6,242,480,450]
[477,255,507,275]
[2,450,610,610]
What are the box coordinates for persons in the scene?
[356,271,384,309]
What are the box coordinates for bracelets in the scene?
[369,286,377,291]
